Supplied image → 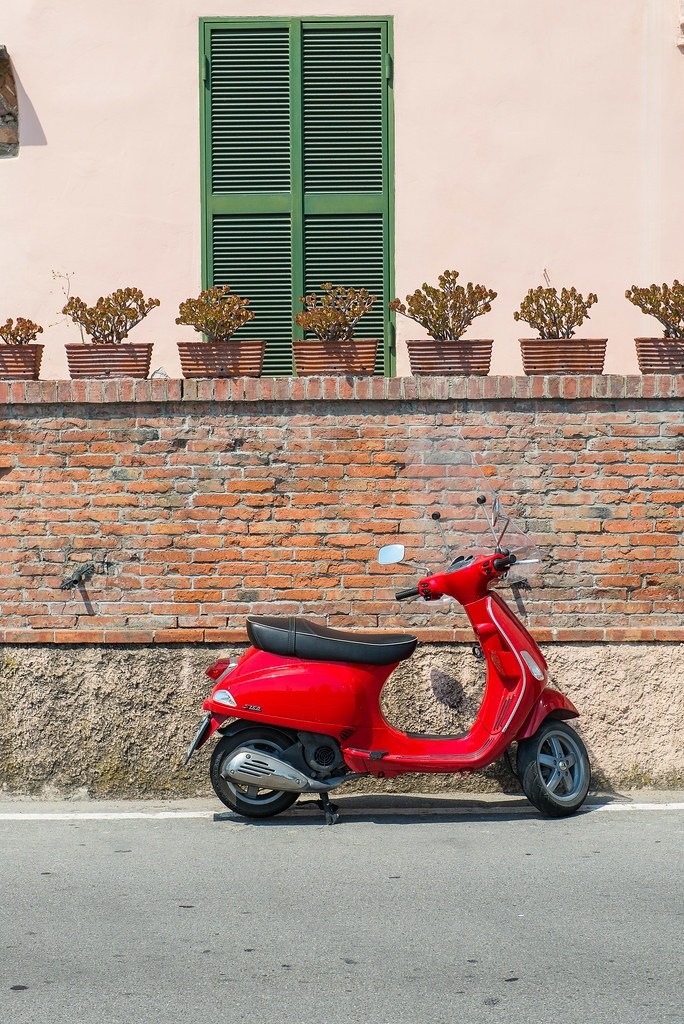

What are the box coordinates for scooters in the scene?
[183,495,591,825]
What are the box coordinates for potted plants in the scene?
[176,284,267,378]
[514,286,608,375]
[388,270,498,375]
[62,288,160,379]
[0,318,44,380]
[625,279,684,374]
[293,283,379,376]
